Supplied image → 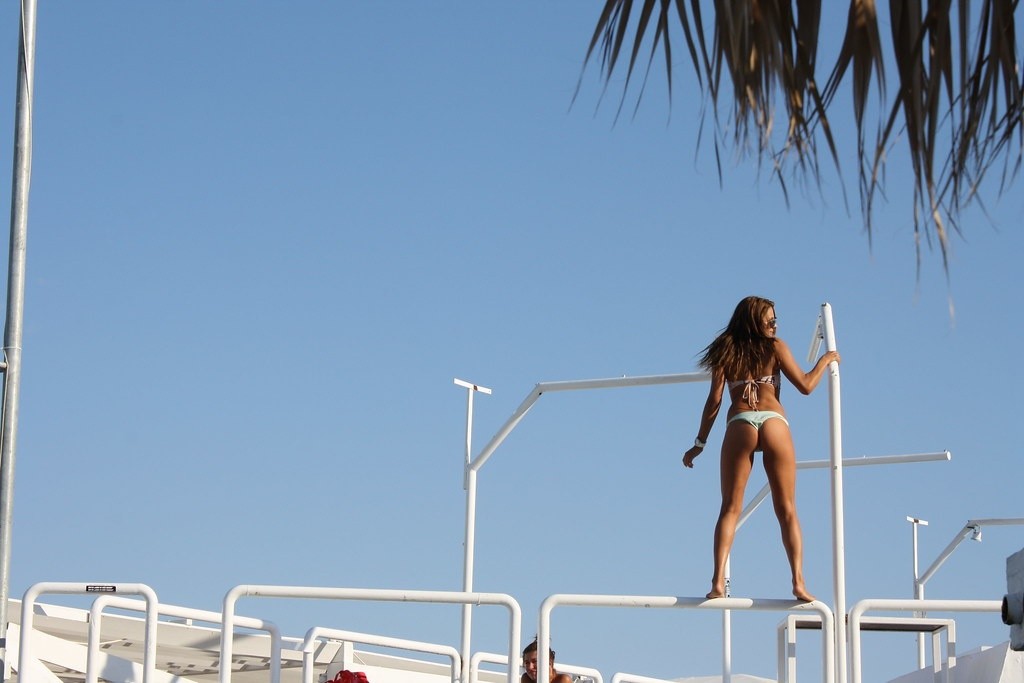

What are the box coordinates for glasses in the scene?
[763,320,777,329]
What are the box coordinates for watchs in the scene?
[693,437,706,448]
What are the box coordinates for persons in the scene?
[682,294,840,603]
[521,631,574,682]
